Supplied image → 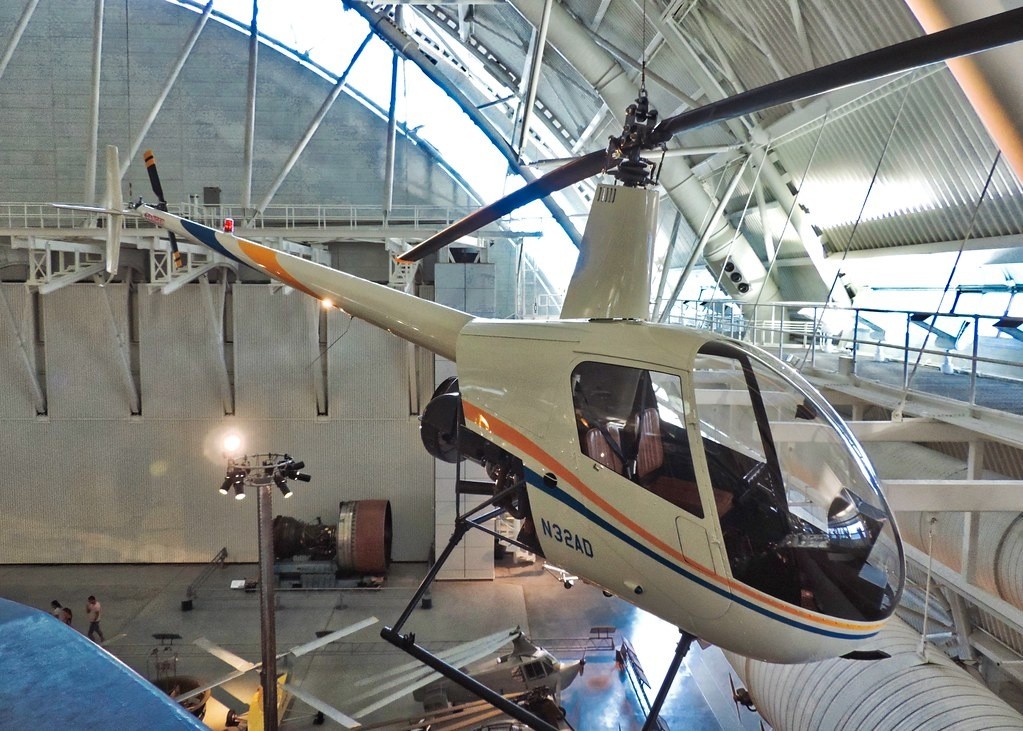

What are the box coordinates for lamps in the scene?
[274,472,293,498]
[233,476,245,500]
[264,463,274,475]
[286,461,305,472]
[794,399,817,420]
[287,471,311,482]
[219,475,233,495]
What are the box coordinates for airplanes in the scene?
[412,627,587,731]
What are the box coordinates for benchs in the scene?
[587,408,734,525]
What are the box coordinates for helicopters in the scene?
[45,6,1022,731]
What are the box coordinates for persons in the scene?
[86,595,105,643]
[49,600,73,626]
